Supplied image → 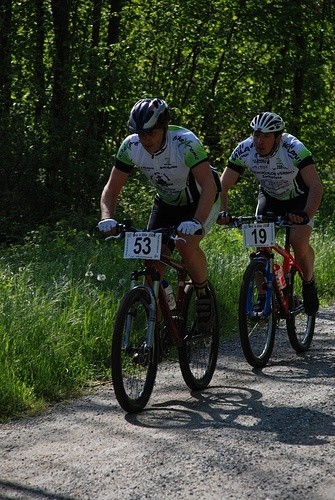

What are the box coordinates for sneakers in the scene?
[195,286,215,330]
[131,353,145,364]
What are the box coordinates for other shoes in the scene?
[301,284,320,315]
[253,296,265,312]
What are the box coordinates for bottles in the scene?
[161,280,176,310]
[274,263,286,289]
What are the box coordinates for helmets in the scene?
[127,97,170,134]
[250,111,285,134]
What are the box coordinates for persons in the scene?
[97,98,223,356]
[216,111,324,317]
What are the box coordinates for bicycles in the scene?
[95,218,221,413]
[219,213,317,370]
